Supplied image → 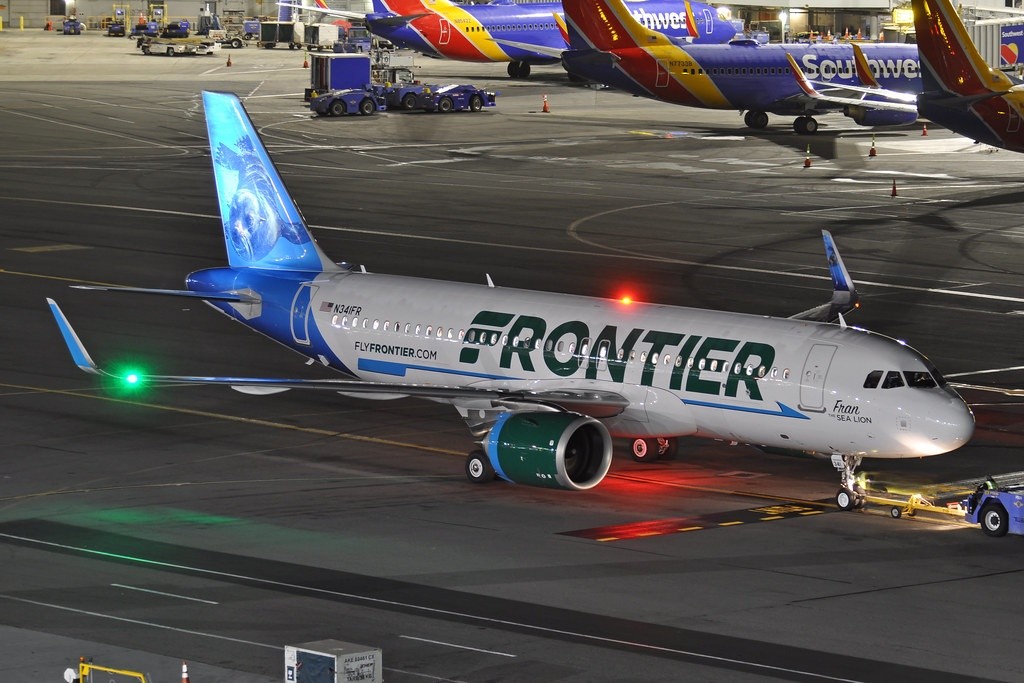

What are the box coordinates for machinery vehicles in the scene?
[62,15,82,35]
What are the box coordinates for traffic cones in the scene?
[920,123,929,136]
[891,179,898,196]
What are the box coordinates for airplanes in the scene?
[47,91,977,509]
[276,0,1024,154]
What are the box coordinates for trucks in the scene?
[137,24,222,56]
[259,20,379,53]
